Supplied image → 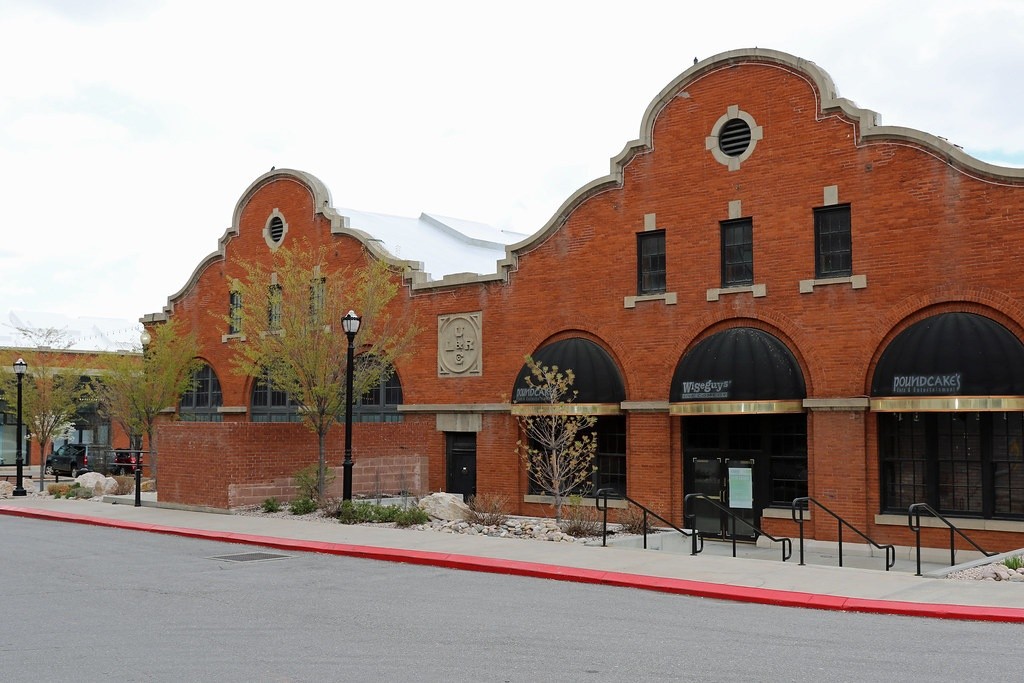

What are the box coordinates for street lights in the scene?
[335,308,364,517]
[134,327,151,507]
[13,357,28,496]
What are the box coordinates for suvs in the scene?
[45,443,118,478]
[110,448,144,476]
